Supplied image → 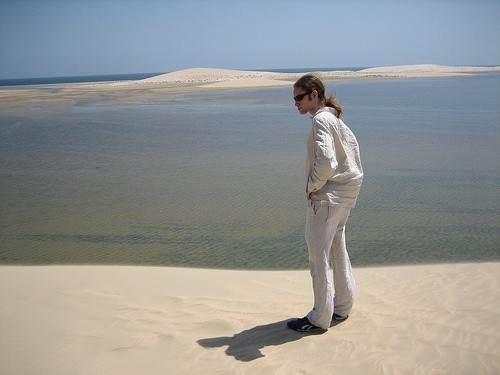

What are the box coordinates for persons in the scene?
[287,74,363,332]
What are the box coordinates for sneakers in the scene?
[333,313,348,321]
[287,316,322,331]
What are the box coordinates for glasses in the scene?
[294,91,312,101]
